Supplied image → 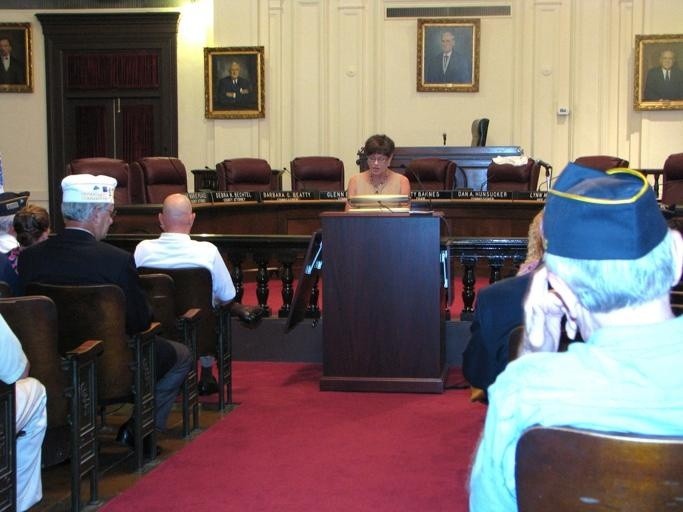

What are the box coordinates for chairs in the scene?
[1,153,236,512]
[468,117,491,146]
[463,155,682,510]
[403,157,469,263]
[213,158,284,268]
[289,156,346,253]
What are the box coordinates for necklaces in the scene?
[369,169,389,193]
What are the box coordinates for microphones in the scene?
[284,168,305,191]
[204,166,241,192]
[480,170,499,192]
[537,160,550,169]
[399,164,432,211]
[443,132,447,146]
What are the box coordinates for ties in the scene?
[234,80,236,88]
[444,56,448,68]
[665,71,669,86]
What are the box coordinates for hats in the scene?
[0,191,30,216]
[540,162,668,260]
[61,174,119,204]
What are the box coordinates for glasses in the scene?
[366,155,388,164]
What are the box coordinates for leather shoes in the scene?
[116,418,160,457]
[198,376,220,396]
[239,305,263,328]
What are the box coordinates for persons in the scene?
[425,31,471,83]
[343,136,410,213]
[644,50,682,101]
[215,62,255,109]
[134,193,237,396]
[1,36,25,82]
[17,174,193,460]
[0,206,50,512]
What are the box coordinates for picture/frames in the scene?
[0,21,36,95]
[631,33,682,112]
[416,16,481,94]
[202,45,267,121]
